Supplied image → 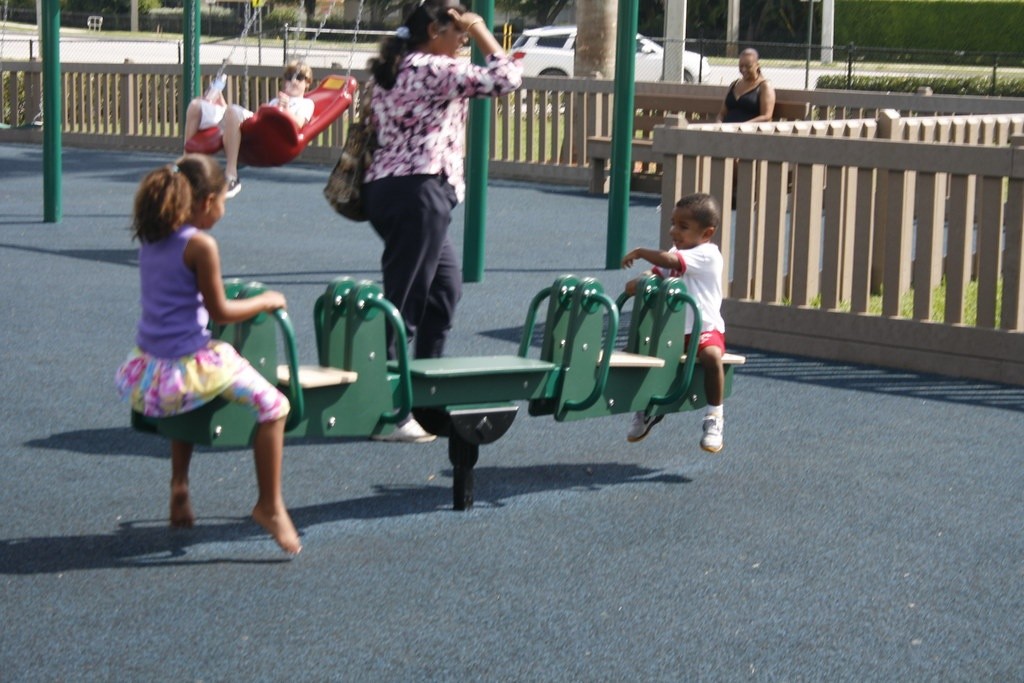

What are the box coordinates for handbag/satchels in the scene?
[322,123,378,222]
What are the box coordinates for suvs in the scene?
[506,24,710,108]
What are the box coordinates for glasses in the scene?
[284,72,307,81]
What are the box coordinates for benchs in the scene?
[586,93,811,197]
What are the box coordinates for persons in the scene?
[113,153,303,556]
[352,0,520,444]
[619,193,728,453]
[716,48,776,209]
[172,59,315,199]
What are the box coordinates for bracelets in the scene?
[465,18,485,34]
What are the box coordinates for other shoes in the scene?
[371,418,436,443]
[224,175,241,199]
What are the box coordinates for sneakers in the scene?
[628,411,665,443]
[700,413,724,453]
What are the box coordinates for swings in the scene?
[181,0,370,169]
